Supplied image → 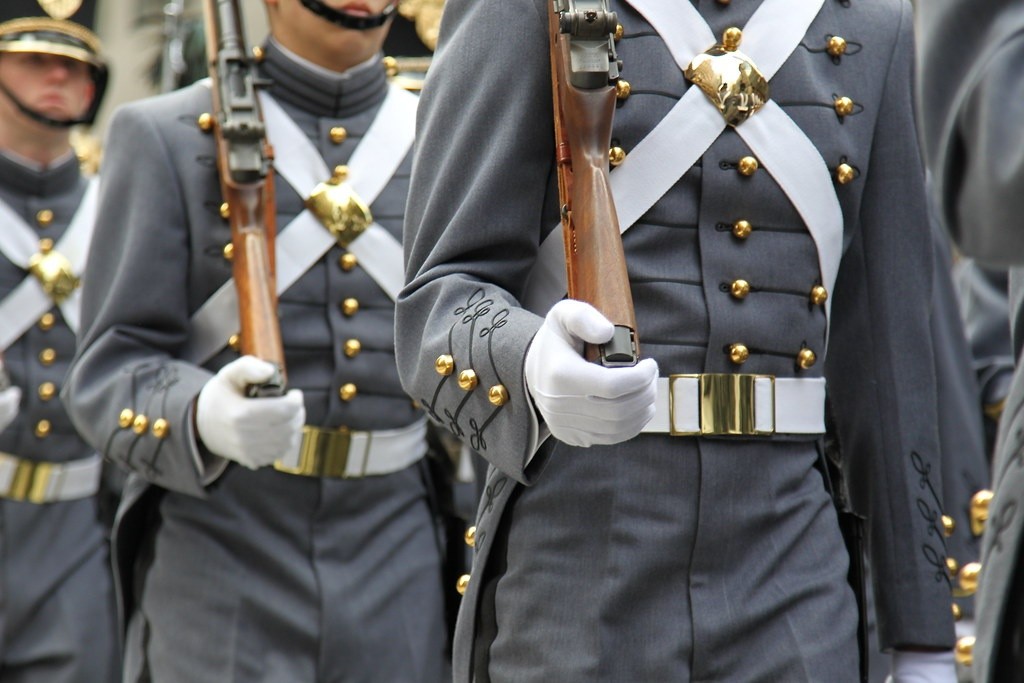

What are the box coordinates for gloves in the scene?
[0,385,22,435]
[890,651,958,683]
[522,300,658,448]
[196,355,306,470]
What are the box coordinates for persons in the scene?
[58,0,452,683]
[393,0,992,683]
[905,0,1024,683]
[0,0,132,683]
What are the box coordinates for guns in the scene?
[547,0,640,368]
[202,0,289,399]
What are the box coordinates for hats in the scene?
[0,0,116,71]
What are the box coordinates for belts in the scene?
[0,452,105,505]
[638,374,827,437]
[271,412,432,480]
